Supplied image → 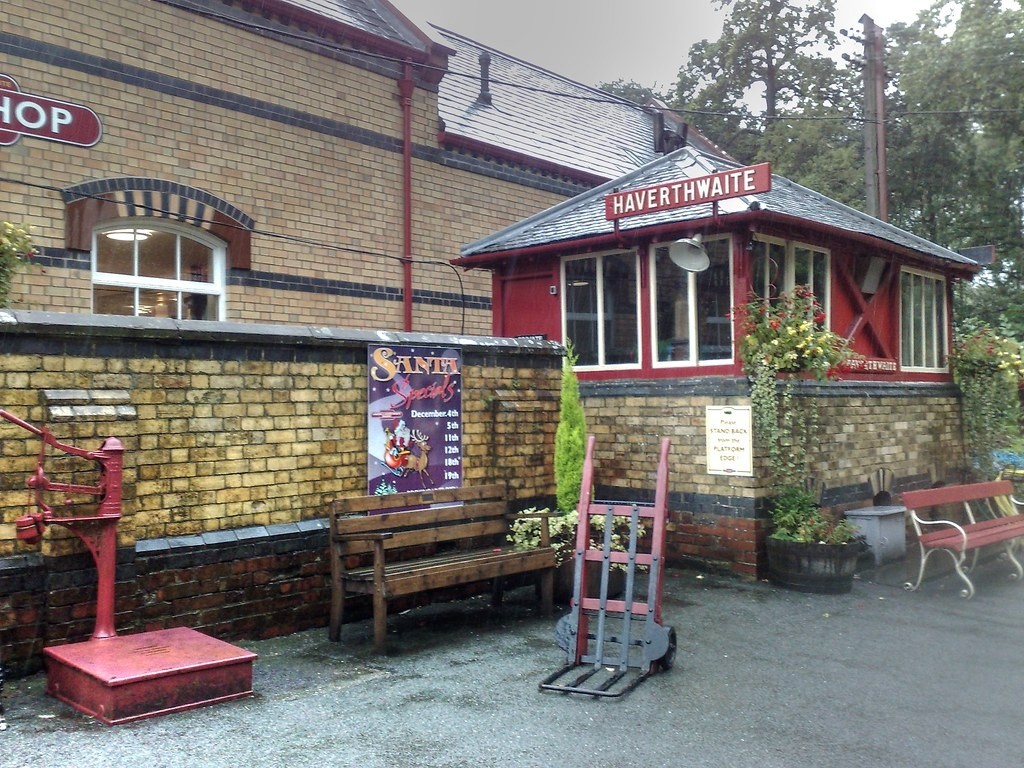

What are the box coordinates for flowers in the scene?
[778,506,858,546]
[965,453,1022,486]
[507,503,648,573]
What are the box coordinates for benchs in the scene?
[901,480,1024,598]
[329,486,563,652]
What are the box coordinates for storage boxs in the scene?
[846,507,905,564]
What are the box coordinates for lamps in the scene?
[668,232,709,271]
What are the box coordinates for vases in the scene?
[769,539,860,594]
[535,540,627,601]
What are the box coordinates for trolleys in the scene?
[537,434,675,701]
